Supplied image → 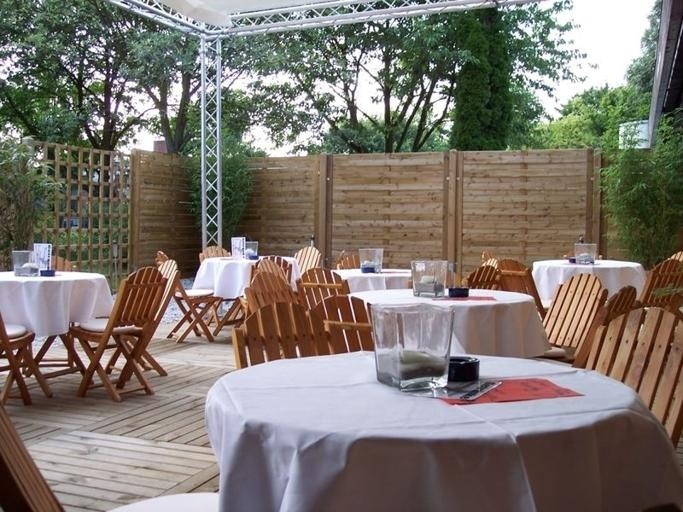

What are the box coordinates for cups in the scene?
[245,241,258,259]
[574,243,597,267]
[12,249,42,278]
[411,261,457,298]
[370,302,454,393]
[359,248,384,274]
